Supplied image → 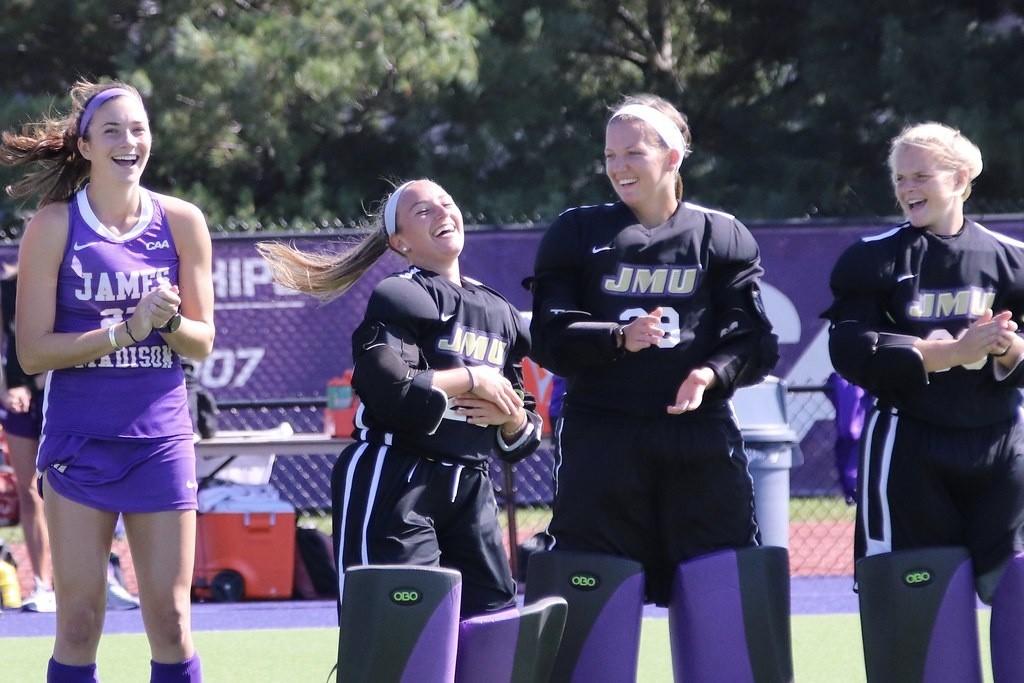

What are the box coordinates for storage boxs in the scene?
[189,511,299,602]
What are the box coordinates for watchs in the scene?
[154,313,181,333]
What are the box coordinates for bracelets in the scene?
[126,320,138,343]
[502,409,527,435]
[988,343,1012,357]
[618,325,626,348]
[109,325,121,351]
[463,366,474,393]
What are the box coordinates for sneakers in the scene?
[21,577,57,613]
[106,585,140,611]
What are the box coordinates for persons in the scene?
[0,82,215,683]
[819,124,1024,683]
[0,270,140,612]
[512,95,795,683]
[257,179,569,683]
